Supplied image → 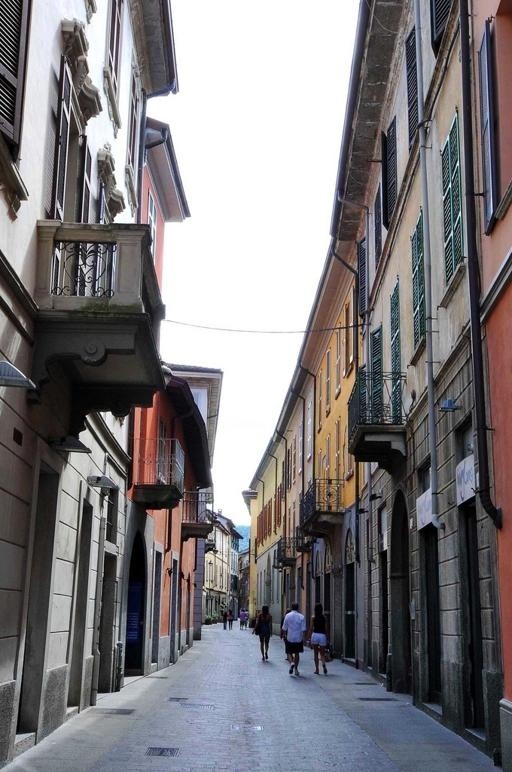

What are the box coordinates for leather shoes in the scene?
[322,666,328,674]
[313,670,319,674]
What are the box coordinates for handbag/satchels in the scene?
[255,623,259,635]
[325,644,334,662]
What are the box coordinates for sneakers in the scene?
[294,669,299,675]
[288,662,296,674]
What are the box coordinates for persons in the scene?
[222,604,295,660]
[306,603,329,674]
[281,602,307,675]
